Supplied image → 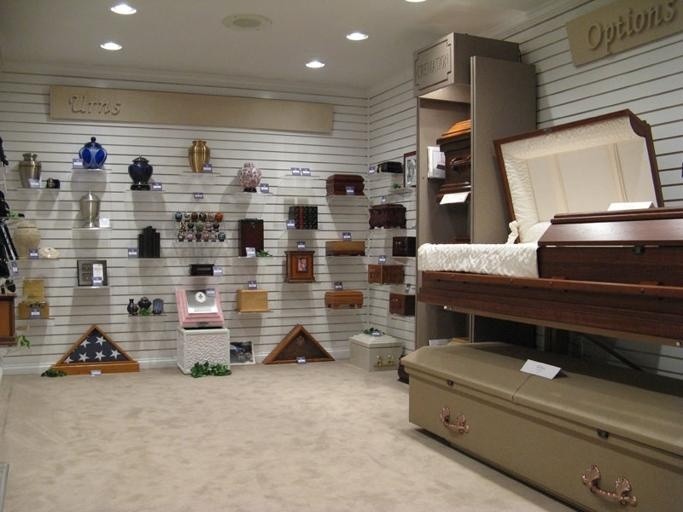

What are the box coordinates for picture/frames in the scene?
[52,323,140,374]
[0,296,18,345]
[229,339,255,365]
[76,259,107,286]
[404,151,417,188]
[263,324,335,365]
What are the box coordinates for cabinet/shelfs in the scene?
[417,57,537,347]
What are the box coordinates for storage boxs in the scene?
[378,162,402,172]
[18,301,49,318]
[389,293,415,316]
[325,290,363,308]
[289,206,317,229]
[413,32,521,102]
[368,264,404,284]
[176,327,230,375]
[237,290,268,312]
[349,332,402,373]
[369,204,406,229]
[393,237,416,257]
[326,175,364,195]
[325,241,365,256]
